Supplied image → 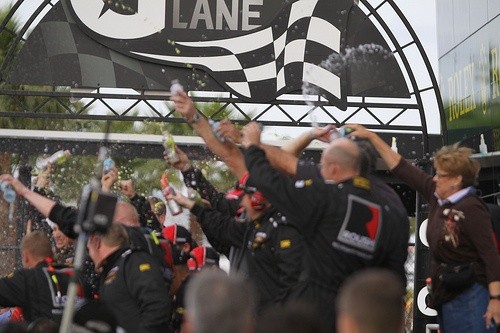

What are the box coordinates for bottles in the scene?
[162,130,180,164]
[390,136,398,154]
[325,128,356,141]
[36,150,72,170]
[426,277,433,296]
[206,116,225,143]
[171,78,184,103]
[103,150,115,176]
[160,177,183,216]
[0,175,16,202]
[479,134,488,156]
[483,313,500,333]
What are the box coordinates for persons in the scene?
[0,88,500,333]
[82,197,181,333]
[342,122,500,333]
[333,268,405,332]
[0,229,88,324]
[179,267,260,333]
[241,115,409,333]
[258,296,334,333]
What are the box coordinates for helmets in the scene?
[223,171,270,210]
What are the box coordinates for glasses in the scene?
[435,171,454,178]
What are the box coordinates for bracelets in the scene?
[186,113,200,125]
[488,295,500,300]
